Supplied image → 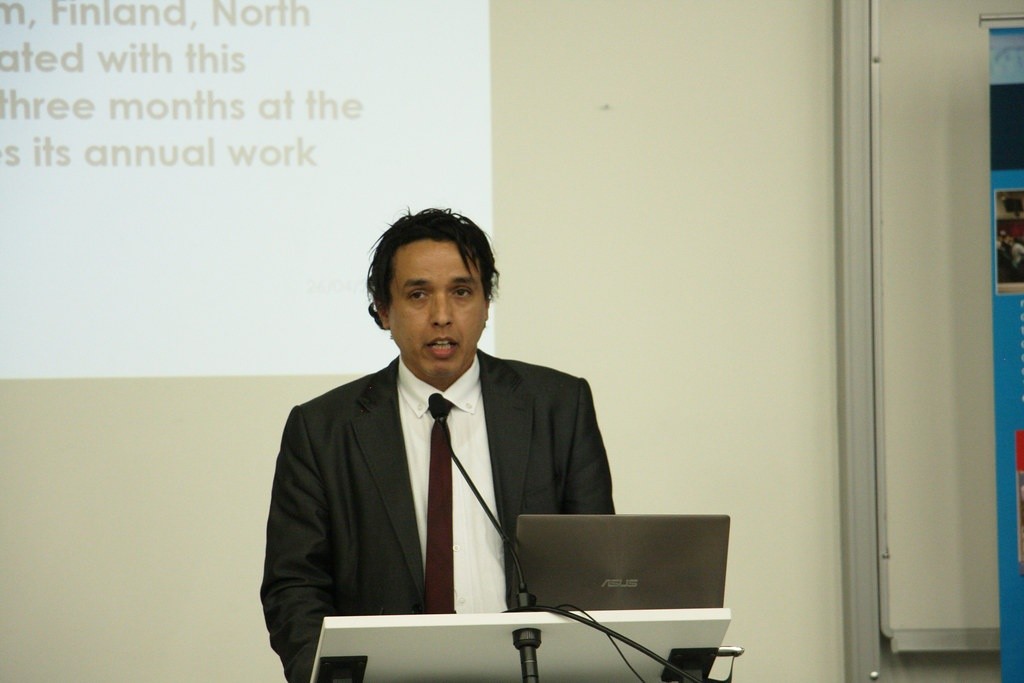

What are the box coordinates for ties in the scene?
[413,398,463,615]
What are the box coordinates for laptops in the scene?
[508,514,728,607]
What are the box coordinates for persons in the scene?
[259,205,616,683]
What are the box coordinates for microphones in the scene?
[427,393,532,611]
